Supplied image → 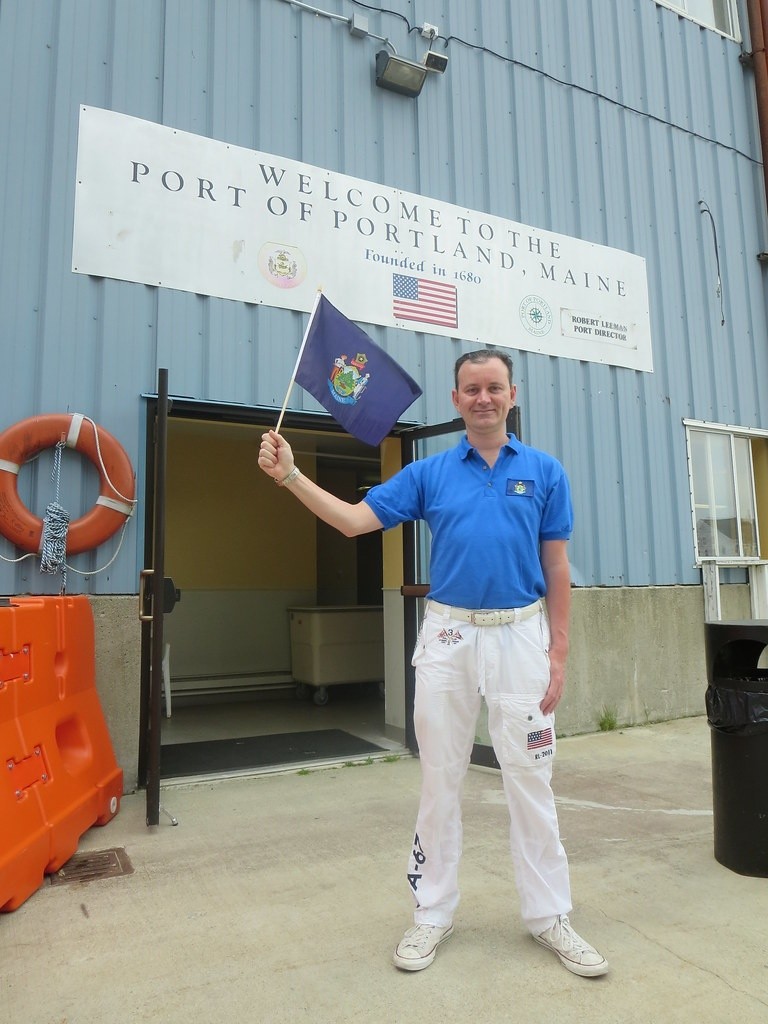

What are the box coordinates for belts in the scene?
[427,599,542,626]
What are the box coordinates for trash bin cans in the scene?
[703,618,767,878]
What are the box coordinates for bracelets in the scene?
[273,466,300,487]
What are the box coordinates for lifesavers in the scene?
[0,414,134,554]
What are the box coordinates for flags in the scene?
[293,293,425,447]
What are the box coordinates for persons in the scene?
[257,349,618,982]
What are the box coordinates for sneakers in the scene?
[532,920,609,977]
[393,923,454,971]
